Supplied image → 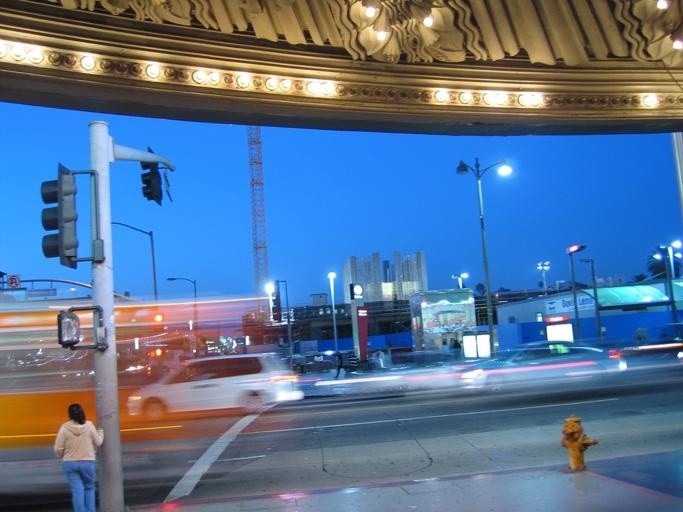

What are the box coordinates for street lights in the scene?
[652,240,683,310]
[140,145,162,208]
[265,282,275,322]
[580,258,603,341]
[555,280,566,293]
[38,162,79,270]
[450,272,469,289]
[165,277,200,336]
[328,271,338,357]
[279,278,293,372]
[457,155,514,360]
[535,259,551,316]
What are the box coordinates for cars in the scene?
[0,336,242,384]
[460,339,628,393]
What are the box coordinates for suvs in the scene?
[123,351,305,424]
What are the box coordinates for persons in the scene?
[334,351,350,380]
[53,403,105,511]
[371,349,385,369]
[451,339,461,360]
[440,341,450,361]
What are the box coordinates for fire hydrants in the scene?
[558,412,599,471]
